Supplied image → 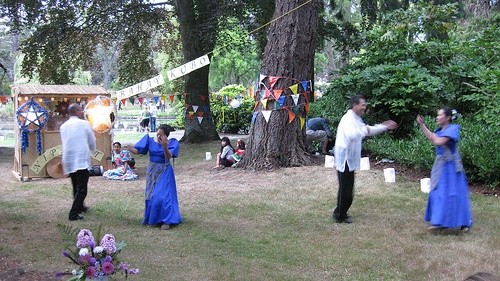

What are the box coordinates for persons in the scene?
[60,104,96,220]
[127,124,183,229]
[112,142,135,168]
[332,95,397,223]
[213,136,245,168]
[306,116,331,154]
[119,89,323,132]
[416,107,472,232]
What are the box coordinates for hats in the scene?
[47,156,70,180]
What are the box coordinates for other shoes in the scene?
[334,218,342,223]
[74,214,83,220]
[161,223,170,230]
[79,206,89,213]
[427,225,447,229]
[212,166,222,170]
[342,217,353,223]
[320,152,330,155]
[220,165,225,169]
[460,221,474,232]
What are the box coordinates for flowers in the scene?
[52,223,139,281]
